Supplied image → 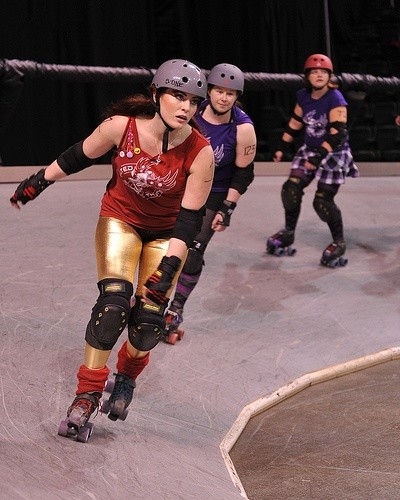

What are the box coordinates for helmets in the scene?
[150,59,207,104]
[304,54,333,74]
[206,63,244,94]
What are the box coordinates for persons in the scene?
[269,54,360,269]
[10,59,216,443]
[130,64,256,345]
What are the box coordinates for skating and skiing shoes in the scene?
[320,241,348,268]
[58,392,104,443]
[99,372,137,421]
[159,301,186,344]
[266,231,297,256]
